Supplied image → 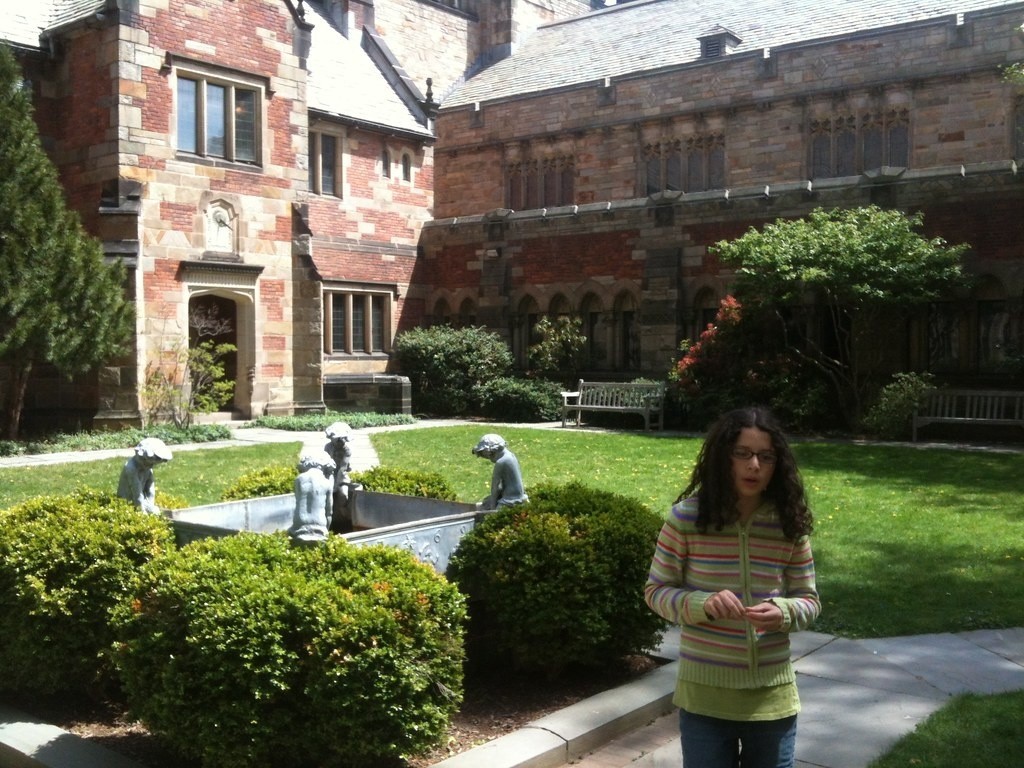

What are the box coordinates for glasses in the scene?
[731,446,779,465]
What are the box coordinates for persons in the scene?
[286,421,355,537]
[116,437,173,517]
[644,407,823,768]
[472,433,529,510]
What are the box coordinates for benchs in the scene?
[559,378,667,432]
[912,387,1024,442]
